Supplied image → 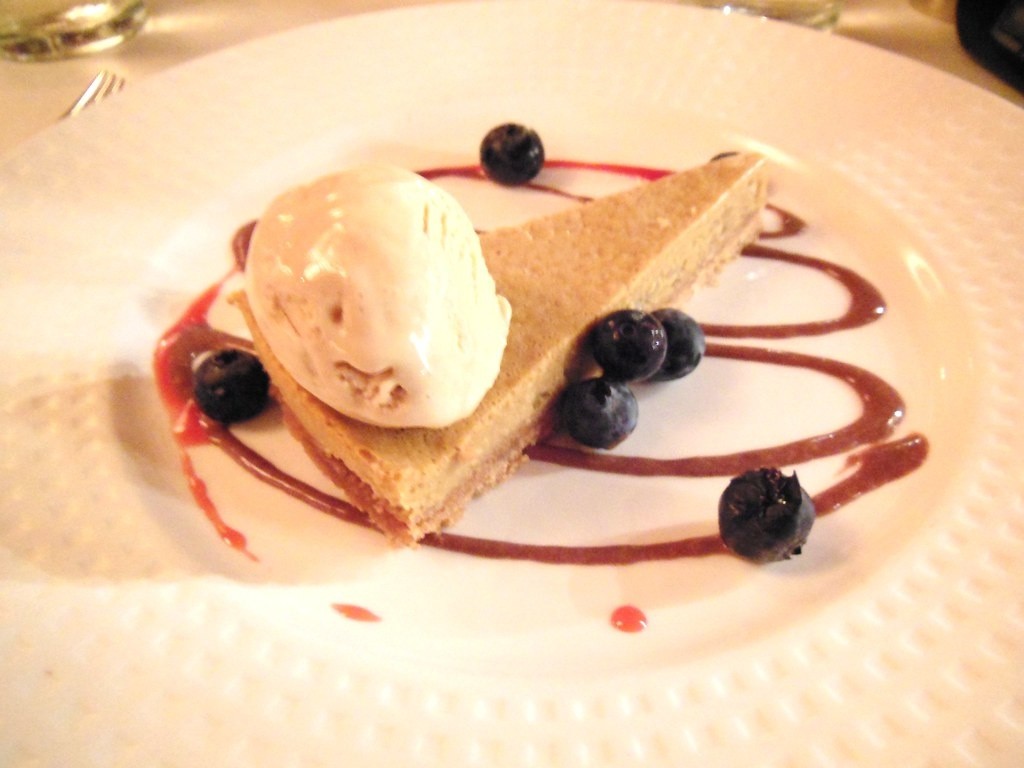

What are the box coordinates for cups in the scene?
[0,0,149,63]
[689,0,842,37]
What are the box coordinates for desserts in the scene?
[232,149,781,546]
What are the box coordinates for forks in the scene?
[63,70,128,116]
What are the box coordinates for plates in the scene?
[0,0,1024,767]
